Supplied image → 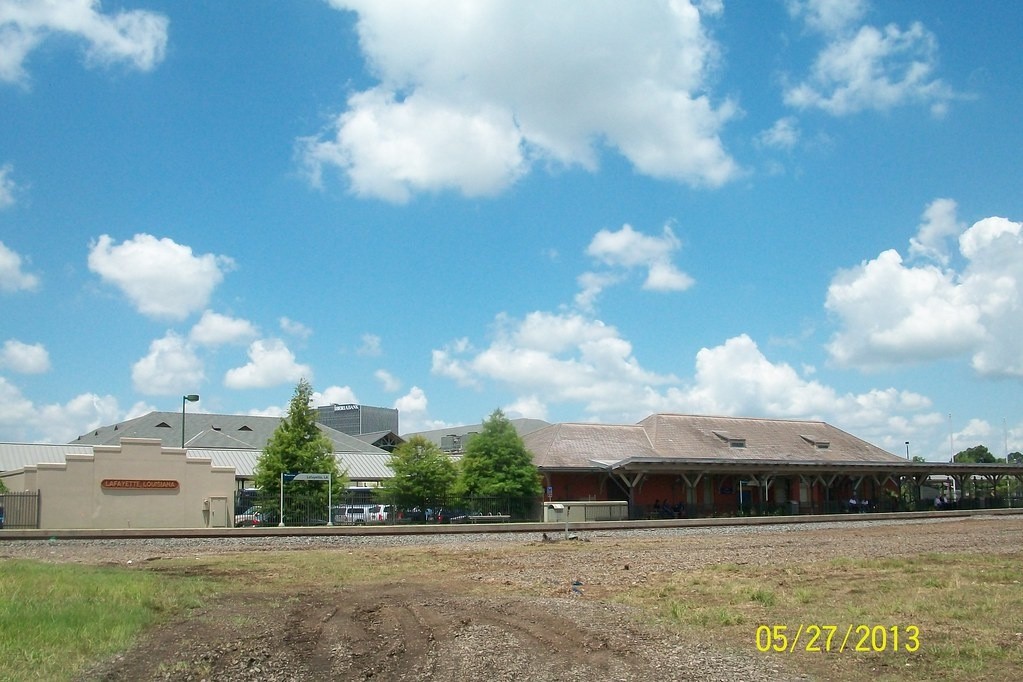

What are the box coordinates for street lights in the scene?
[948,413,956,502]
[1002,416,1011,508]
[182,394,200,449]
[905,441,909,460]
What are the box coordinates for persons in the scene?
[425,509,433,520]
[934,494,948,509]
[848,495,868,513]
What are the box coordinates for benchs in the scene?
[470,514,511,523]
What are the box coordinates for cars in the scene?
[251,507,328,527]
[234,505,263,528]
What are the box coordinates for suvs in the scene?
[331,503,452,527]
